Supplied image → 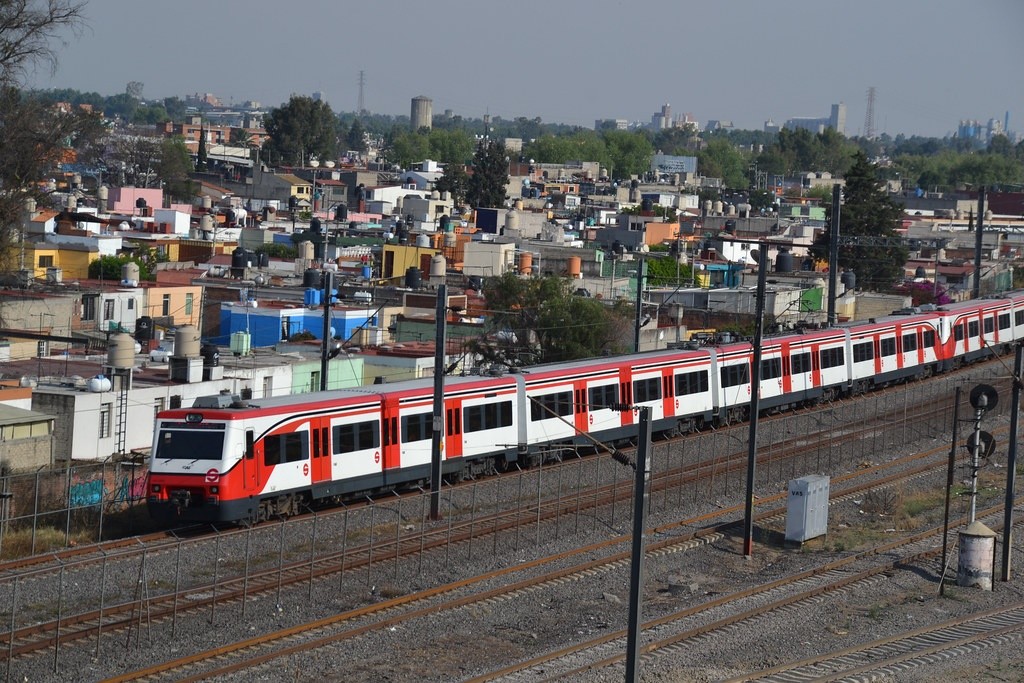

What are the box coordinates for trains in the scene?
[140,293,1024,530]
